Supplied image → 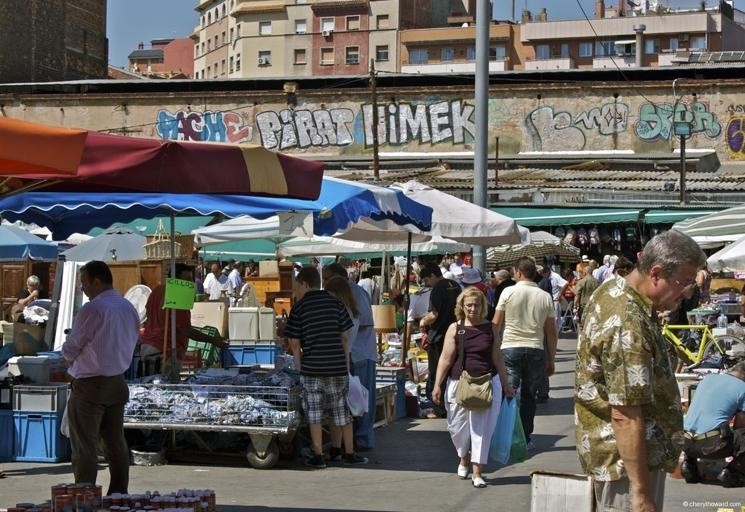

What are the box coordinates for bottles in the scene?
[729,288,736,301]
[717,314,728,329]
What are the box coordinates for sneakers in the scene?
[426,409,446,418]
[717,469,744,487]
[526,437,537,449]
[538,394,548,403]
[681,460,699,483]
[470,472,486,487]
[305,441,372,469]
[457,450,471,480]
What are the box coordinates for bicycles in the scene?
[657,307,745,374]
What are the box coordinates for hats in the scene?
[582,255,589,262]
[462,268,483,284]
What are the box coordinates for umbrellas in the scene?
[331,178,522,368]
[672,203,745,274]
[0,175,434,384]
[1,115,327,202]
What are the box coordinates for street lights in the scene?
[673,120,694,206]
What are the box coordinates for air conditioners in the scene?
[257,57,266,65]
[322,30,332,37]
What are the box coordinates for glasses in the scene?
[671,274,697,293]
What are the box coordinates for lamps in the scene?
[371,305,398,366]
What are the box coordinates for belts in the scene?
[683,430,719,442]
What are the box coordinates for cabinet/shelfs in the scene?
[241,273,293,305]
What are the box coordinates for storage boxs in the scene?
[0,356,71,462]
[191,301,281,365]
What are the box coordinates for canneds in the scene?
[51,482,102,511]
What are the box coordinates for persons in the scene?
[491,256,576,450]
[572,228,707,511]
[573,253,634,324]
[195,257,258,307]
[655,260,712,364]
[276,256,379,469]
[431,286,516,489]
[390,254,500,418]
[16,274,41,324]
[681,361,745,488]
[60,259,141,496]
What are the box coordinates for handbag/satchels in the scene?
[609,227,636,251]
[455,370,494,408]
[555,227,600,246]
